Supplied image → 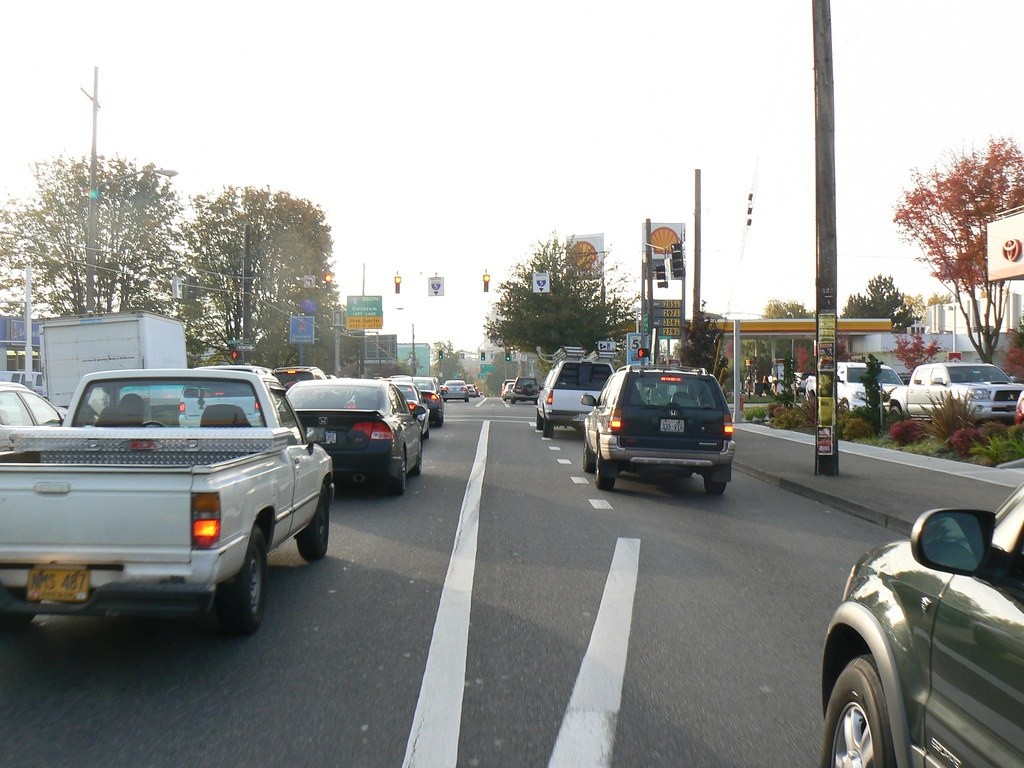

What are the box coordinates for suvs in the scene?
[536,357,736,496]
[511,377,542,404]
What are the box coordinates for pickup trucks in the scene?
[0,368,336,639]
[878,362,1024,423]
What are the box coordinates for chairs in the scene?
[201,404,250,427]
[670,392,699,406]
[99,404,143,425]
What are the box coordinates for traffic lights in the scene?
[395,277,401,293]
[670,243,685,277]
[481,352,485,361]
[439,350,443,359]
[505,349,510,361]
[325,274,332,292]
[638,348,648,358]
[654,262,669,288]
[483,274,490,293]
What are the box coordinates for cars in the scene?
[820,481,1024,768]
[0,363,477,502]
[806,361,904,413]
[500,379,515,402]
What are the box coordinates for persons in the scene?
[759,372,771,397]
[117,394,145,425]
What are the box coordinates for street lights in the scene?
[944,304,957,352]
[85,169,178,314]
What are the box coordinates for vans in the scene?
[793,372,812,396]
